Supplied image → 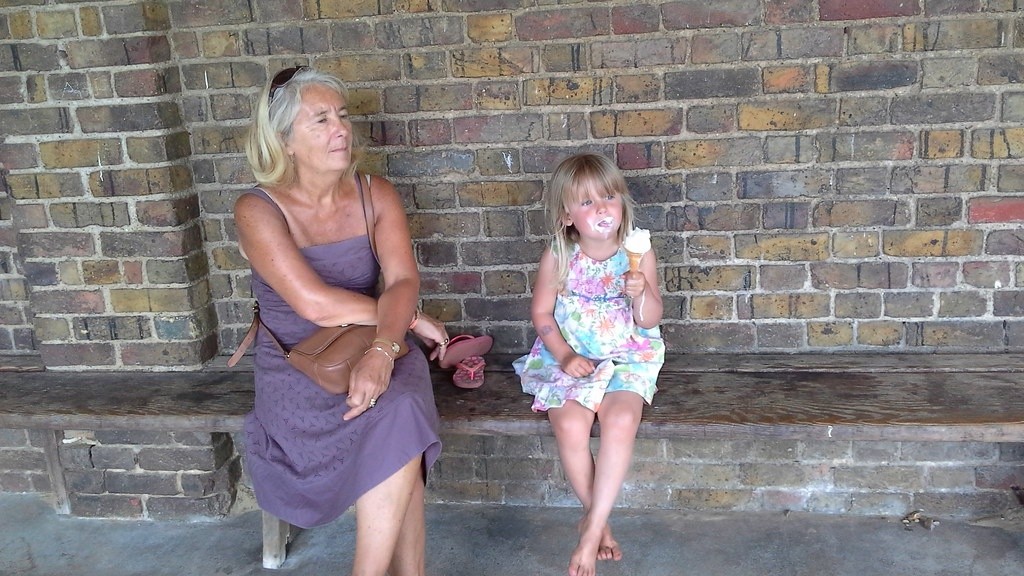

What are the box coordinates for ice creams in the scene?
[622,227,651,274]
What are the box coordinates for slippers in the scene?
[438,335,493,369]
[452,355,486,389]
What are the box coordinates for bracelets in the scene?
[364,347,395,369]
[409,309,422,329]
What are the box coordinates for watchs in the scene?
[372,338,401,356]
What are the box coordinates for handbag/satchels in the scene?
[286,321,411,395]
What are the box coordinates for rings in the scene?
[440,339,448,345]
[369,399,375,407]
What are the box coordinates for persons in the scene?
[513,152,665,576]
[234,65,450,576]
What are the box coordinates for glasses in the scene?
[269,64,310,103]
